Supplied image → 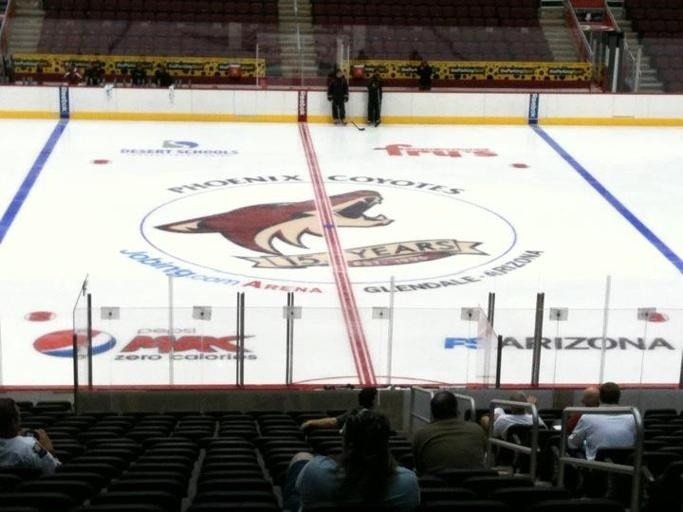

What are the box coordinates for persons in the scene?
[417,60,433,90]
[298,387,379,431]
[326,68,349,124]
[566,385,600,433]
[480,391,545,439]
[356,50,368,60]
[366,70,383,124]
[566,381,644,462]
[287,409,422,512]
[0,397,62,477]
[412,390,488,476]
[63,57,173,91]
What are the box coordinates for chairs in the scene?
[0,0,560,64]
[625,0,683,95]
[0,400,683,512]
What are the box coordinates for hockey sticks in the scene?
[350,120,364,131]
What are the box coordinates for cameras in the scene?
[24,430,37,439]
